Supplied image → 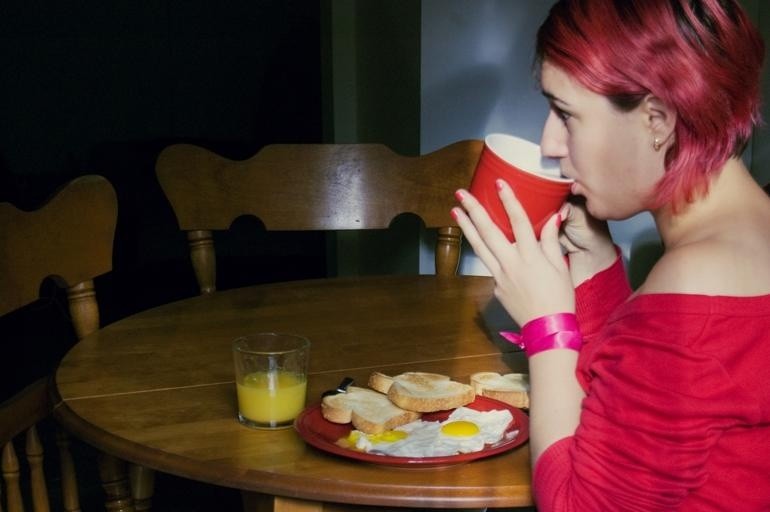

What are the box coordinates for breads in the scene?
[470,371,529,410]
[320,386,422,433]
[367,372,475,413]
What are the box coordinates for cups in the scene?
[229,332,313,424]
[234,427,302,478]
[467,132,575,249]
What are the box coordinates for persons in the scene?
[450,0,770,511]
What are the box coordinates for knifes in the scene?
[318,374,357,400]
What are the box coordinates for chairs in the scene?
[151,125,497,305]
[0,171,163,511]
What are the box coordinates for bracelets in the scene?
[498,314,581,357]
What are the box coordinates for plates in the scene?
[291,397,530,468]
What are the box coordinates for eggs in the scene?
[347,406,519,459]
